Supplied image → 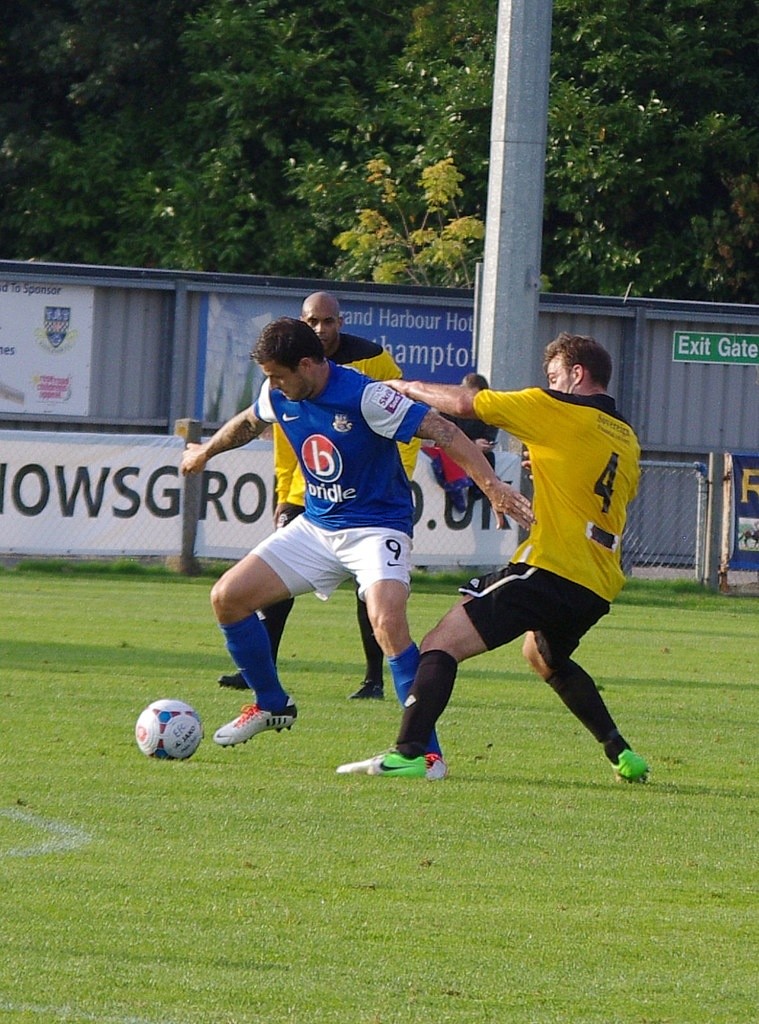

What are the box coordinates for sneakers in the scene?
[347,680,384,699]
[424,754,447,780]
[336,746,426,777]
[217,666,277,689]
[212,695,298,748]
[609,749,650,784]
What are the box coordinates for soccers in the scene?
[135,698,202,762]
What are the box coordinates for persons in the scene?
[179,317,538,781]
[432,371,500,454]
[337,330,651,785]
[219,292,424,701]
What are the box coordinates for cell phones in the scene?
[489,440,498,445]
